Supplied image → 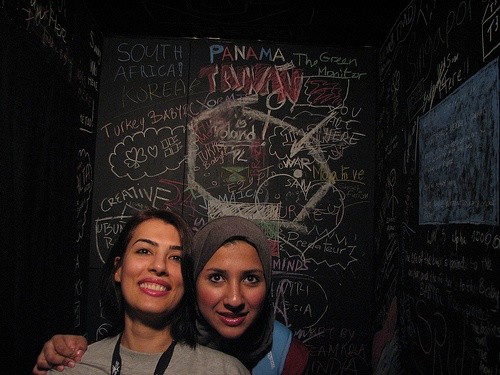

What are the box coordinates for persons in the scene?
[32,216,312,375]
[46,208,251,375]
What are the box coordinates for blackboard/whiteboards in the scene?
[374,0,499,374]
[70,36,374,375]
